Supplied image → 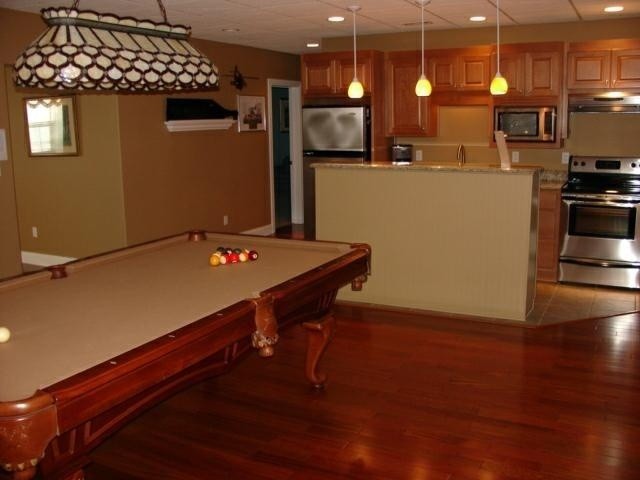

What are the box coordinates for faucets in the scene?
[456,143,466,163]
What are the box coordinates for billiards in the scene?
[1,327,10,342]
[210,247,257,266]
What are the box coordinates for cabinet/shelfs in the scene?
[428,44,492,90]
[487,43,563,98]
[536,183,563,285]
[300,49,377,96]
[384,44,430,136]
[562,39,640,94]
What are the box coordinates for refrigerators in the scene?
[300,104,370,243]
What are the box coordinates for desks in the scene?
[0,228,372,480]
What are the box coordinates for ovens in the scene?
[557,201,640,290]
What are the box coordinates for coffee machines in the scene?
[392,143,413,165]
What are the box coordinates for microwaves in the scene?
[495,107,556,142]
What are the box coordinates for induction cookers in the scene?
[559,172,640,204]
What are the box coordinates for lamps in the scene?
[490,0,508,96]
[12,0,220,98]
[347,5,365,99]
[414,0,433,97]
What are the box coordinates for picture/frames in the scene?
[236,94,267,134]
[279,98,289,133]
[22,94,81,158]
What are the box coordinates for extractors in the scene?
[567,94,640,117]
